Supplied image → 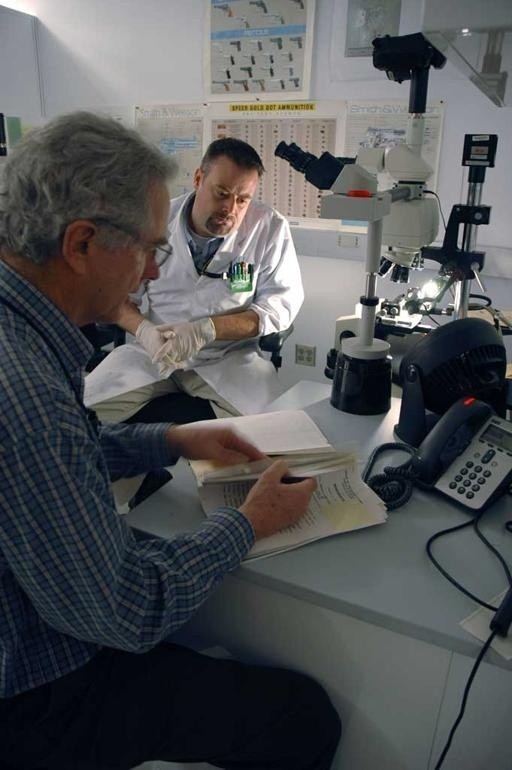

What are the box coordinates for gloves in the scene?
[135,316,217,380]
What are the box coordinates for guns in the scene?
[212,0,304,91]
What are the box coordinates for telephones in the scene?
[412,396,512,514]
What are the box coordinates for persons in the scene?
[1,110,340,769]
[80,137,309,511]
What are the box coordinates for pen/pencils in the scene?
[228,261,253,282]
[280,476,307,484]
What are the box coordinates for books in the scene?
[180,408,389,566]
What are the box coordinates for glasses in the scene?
[91,216,173,269]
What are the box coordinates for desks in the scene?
[117,377,509,670]
